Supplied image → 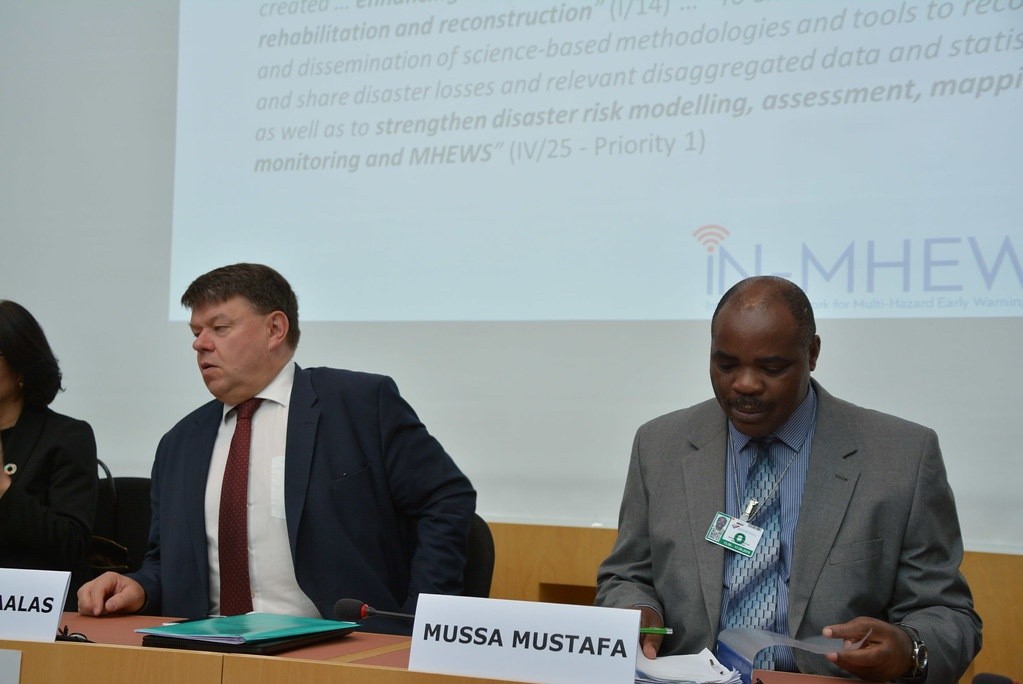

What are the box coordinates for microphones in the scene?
[334,599,414,621]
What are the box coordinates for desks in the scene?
[0,613,532,684]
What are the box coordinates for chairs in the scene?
[89,477,151,563]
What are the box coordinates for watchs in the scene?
[896,625,928,681]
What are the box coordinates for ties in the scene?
[220,397,263,616]
[723,433,778,672]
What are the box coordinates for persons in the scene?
[593,277,986,684]
[714,517,727,532]
[77,263,477,634]
[0,299,98,610]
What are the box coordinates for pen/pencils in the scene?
[710,660,723,674]
[640,627,673,635]
